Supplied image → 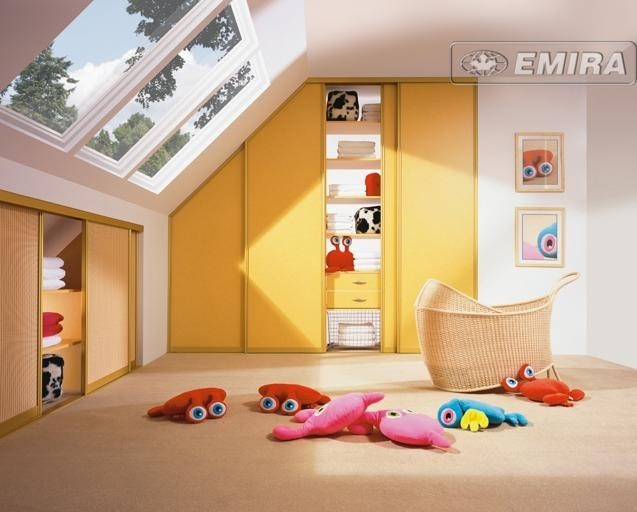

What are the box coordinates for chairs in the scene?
[411,272,584,393]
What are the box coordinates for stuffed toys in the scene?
[325,236,355,273]
[437,398,529,432]
[258,383,330,416]
[347,407,450,447]
[502,364,585,407]
[272,391,384,441]
[147,387,227,424]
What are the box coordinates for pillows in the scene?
[43,255,68,290]
[42,311,64,348]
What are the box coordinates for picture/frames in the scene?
[513,130,568,270]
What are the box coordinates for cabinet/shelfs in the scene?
[41,289,83,354]
[170,72,481,353]
